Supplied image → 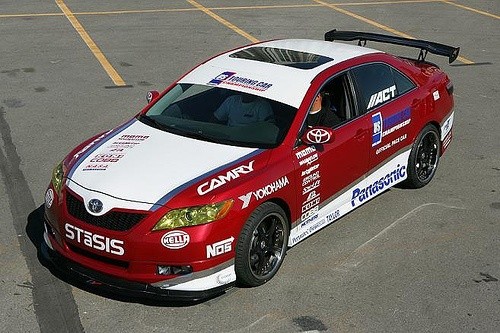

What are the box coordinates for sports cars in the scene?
[43,28,461,305]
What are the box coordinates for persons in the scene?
[209,93,275,126]
[301,88,341,134]
